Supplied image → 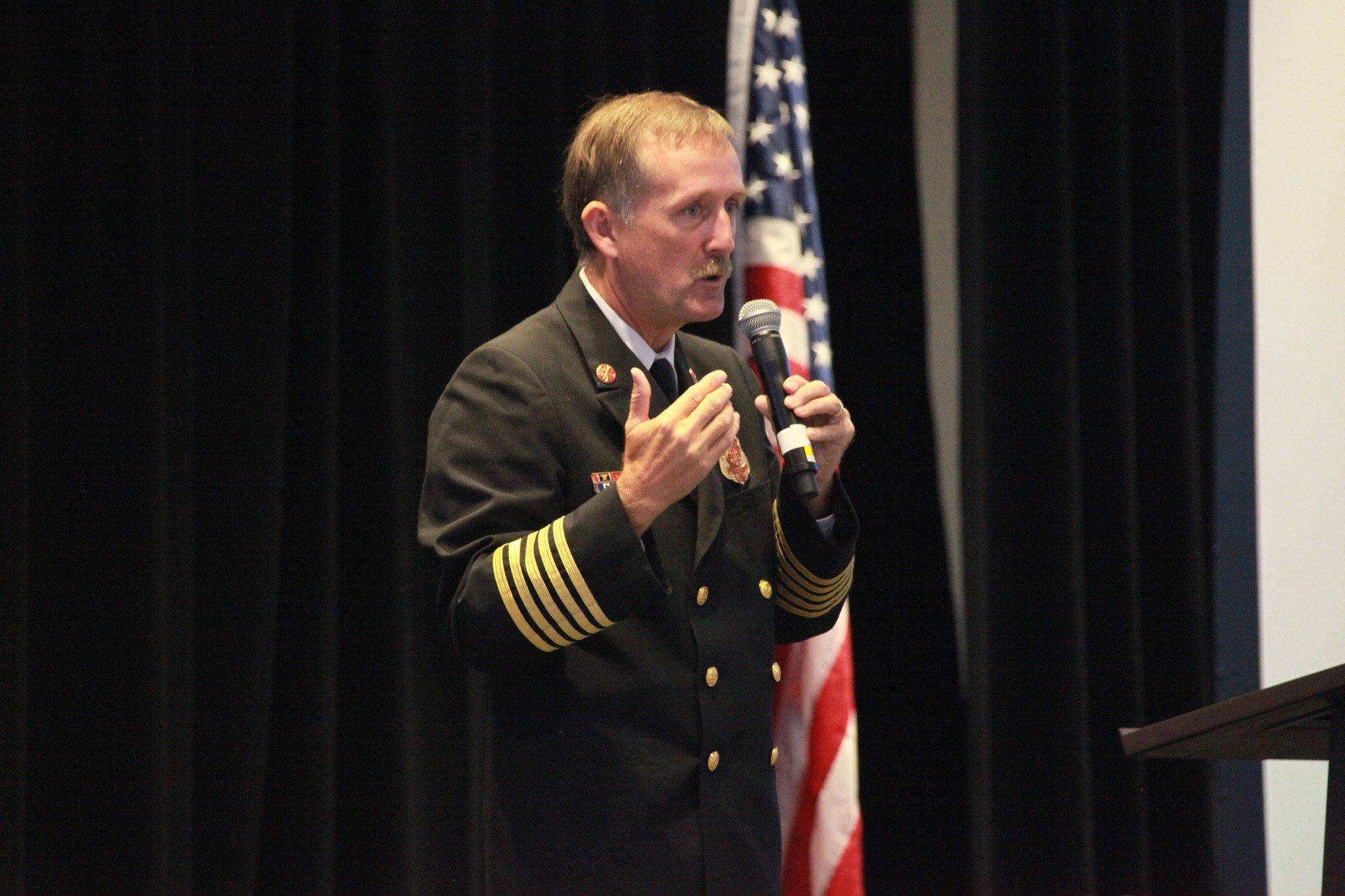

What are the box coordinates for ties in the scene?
[652,356,679,405]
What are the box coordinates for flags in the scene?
[726,0,867,896]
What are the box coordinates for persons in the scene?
[418,90,858,896]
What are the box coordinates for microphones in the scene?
[737,299,821,498]
